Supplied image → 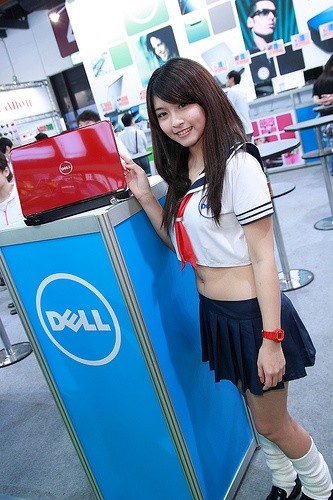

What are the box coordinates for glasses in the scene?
[250,9,278,19]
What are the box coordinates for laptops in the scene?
[10,119,133,226]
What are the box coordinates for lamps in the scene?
[49,6,66,23]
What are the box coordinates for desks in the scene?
[132,151,152,160]
[284,114,333,230]
[255,138,314,292]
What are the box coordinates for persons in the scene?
[117,113,152,177]
[223,70,254,146]
[119,58,333,500]
[0,111,131,229]
[312,54,333,105]
[245,0,277,54]
[146,26,179,69]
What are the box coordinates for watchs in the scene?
[260,329,285,342]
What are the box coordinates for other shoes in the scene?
[299,488,333,500]
[266,472,302,500]
